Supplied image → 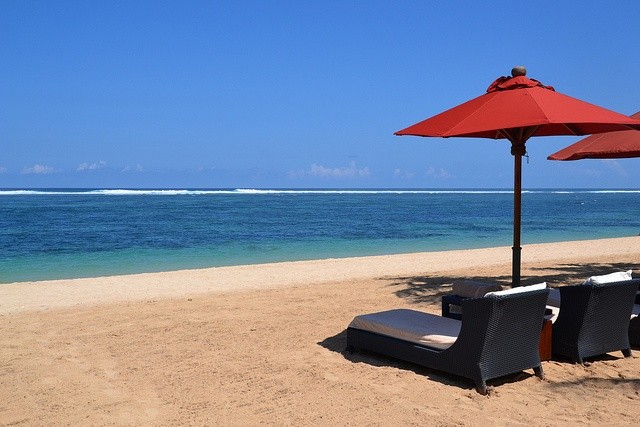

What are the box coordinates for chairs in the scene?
[634,291,640,305]
[347,288,545,394]
[547,288,639,364]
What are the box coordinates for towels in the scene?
[452,280,503,298]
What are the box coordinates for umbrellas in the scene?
[548,113,637,160]
[393,65,637,287]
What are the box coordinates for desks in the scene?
[441,294,552,362]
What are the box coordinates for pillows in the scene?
[581,269,634,285]
[484,281,547,298]
